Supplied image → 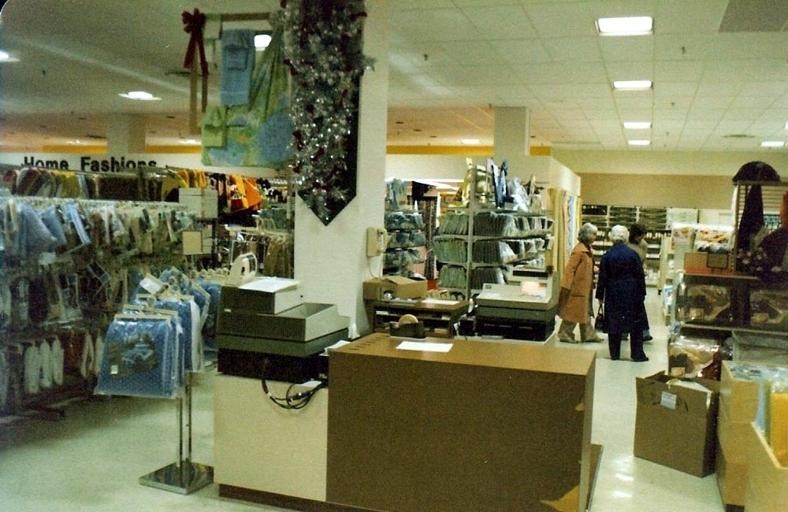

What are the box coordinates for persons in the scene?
[596,224,649,361]
[558,222,604,343]
[621,223,652,341]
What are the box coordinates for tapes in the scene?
[399,314,418,325]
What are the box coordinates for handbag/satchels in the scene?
[594,306,604,330]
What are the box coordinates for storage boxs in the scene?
[630,337,787,511]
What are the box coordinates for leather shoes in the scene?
[611,356,620,360]
[560,335,602,343]
[643,335,652,342]
[636,356,648,361]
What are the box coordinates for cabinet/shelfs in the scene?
[224,155,787,360]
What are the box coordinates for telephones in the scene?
[366,226,387,257]
